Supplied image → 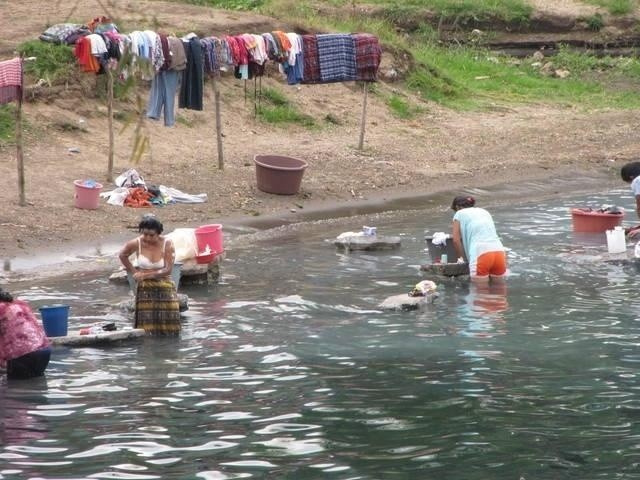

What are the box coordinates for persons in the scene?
[119,217,179,341]
[461,277,510,361]
[0,291,53,379]
[452,195,506,277]
[620,163,640,237]
[0,373,49,455]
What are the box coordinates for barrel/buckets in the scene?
[194,224,225,255]
[39,304,70,337]
[424,234,462,263]
[73,179,104,210]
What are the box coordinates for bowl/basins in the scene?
[191,249,220,264]
[571,208,625,233]
[254,154,309,195]
